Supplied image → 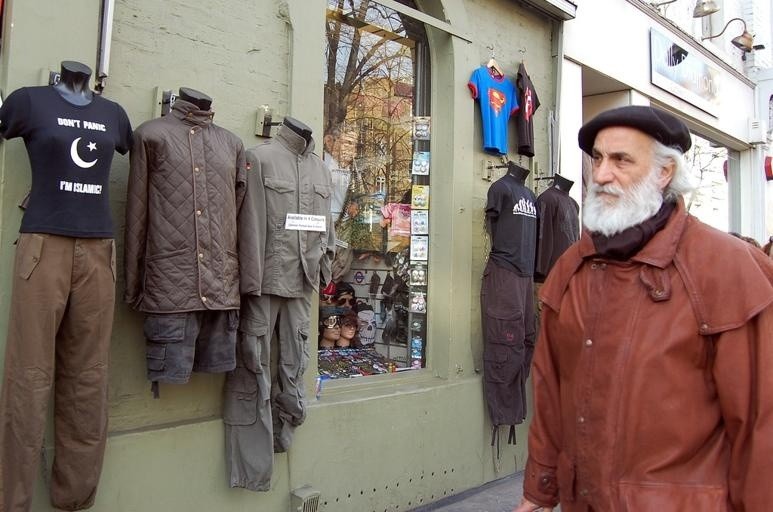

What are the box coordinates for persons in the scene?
[1,60,134,510]
[514,107,772,511]
[126,87,247,383]
[536,173,580,280]
[319,280,375,349]
[222,115,337,492]
[323,123,359,232]
[478,162,540,425]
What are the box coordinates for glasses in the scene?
[323,316,343,328]
[336,296,355,306]
[318,294,337,304]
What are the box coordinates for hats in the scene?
[578,105,692,159]
[319,307,343,321]
[319,281,336,295]
[337,281,355,295]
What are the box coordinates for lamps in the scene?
[650,0,719,22]
[741,44,765,61]
[701,18,753,61]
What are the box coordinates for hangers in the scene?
[519,52,530,77]
[484,48,503,79]
